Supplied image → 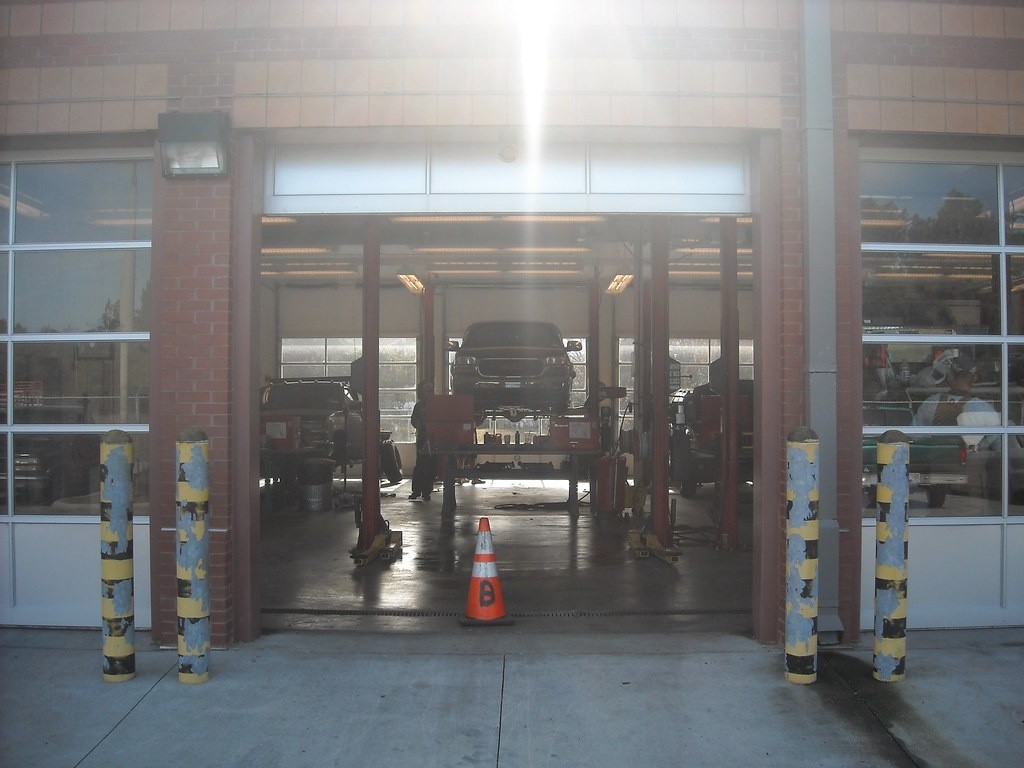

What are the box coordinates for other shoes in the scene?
[471,480,485,484]
[409,492,421,499]
[422,494,431,501]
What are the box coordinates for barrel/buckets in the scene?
[298,458,335,511]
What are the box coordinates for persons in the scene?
[455,454,485,484]
[408,381,435,500]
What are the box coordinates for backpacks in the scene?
[933,392,972,426]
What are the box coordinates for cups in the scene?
[505,435,510,444]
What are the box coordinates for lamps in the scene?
[157,110,235,177]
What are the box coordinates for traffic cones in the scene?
[457,516,515,625]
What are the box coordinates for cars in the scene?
[260,376,363,464]
[861,340,1024,508]
[0,405,101,504]
[444,319,583,421]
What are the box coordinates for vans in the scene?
[667,378,754,497]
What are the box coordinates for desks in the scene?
[432,441,601,520]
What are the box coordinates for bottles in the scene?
[515,431,520,444]
